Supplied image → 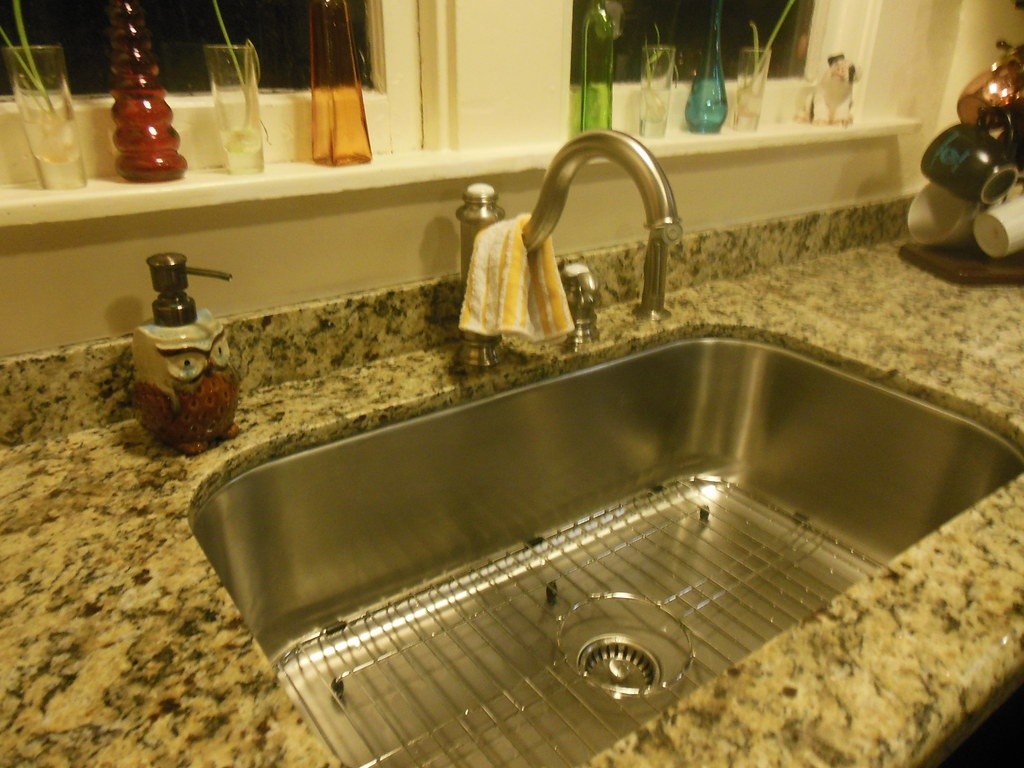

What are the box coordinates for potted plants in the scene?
[0,0,87,190]
[640,23,679,138]
[202,0,271,175]
[732,0,798,134]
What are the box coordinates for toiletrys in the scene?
[132,252,242,457]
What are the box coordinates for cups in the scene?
[906,40,1024,259]
[640,45,676,138]
[734,49,771,131]
[203,44,264,174]
[3,46,87,188]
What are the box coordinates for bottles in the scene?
[684,0,728,133]
[579,0,614,131]
[308,0,373,167]
[104,0,188,180]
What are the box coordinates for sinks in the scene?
[186,322,1024,768]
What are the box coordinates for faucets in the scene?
[453,128,682,367]
